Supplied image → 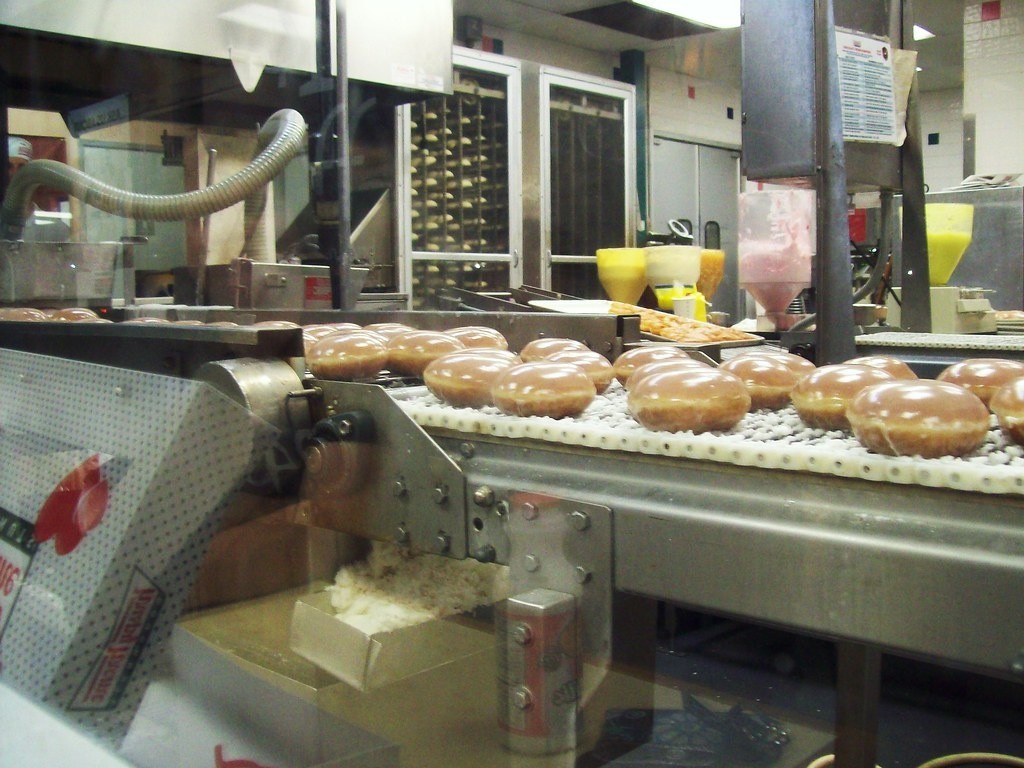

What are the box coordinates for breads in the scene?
[0,306,1023,459]
[411,111,489,296]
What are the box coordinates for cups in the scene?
[671,296,695,321]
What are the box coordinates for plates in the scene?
[995,319,1024,336]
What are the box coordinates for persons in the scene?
[8,136,43,217]
